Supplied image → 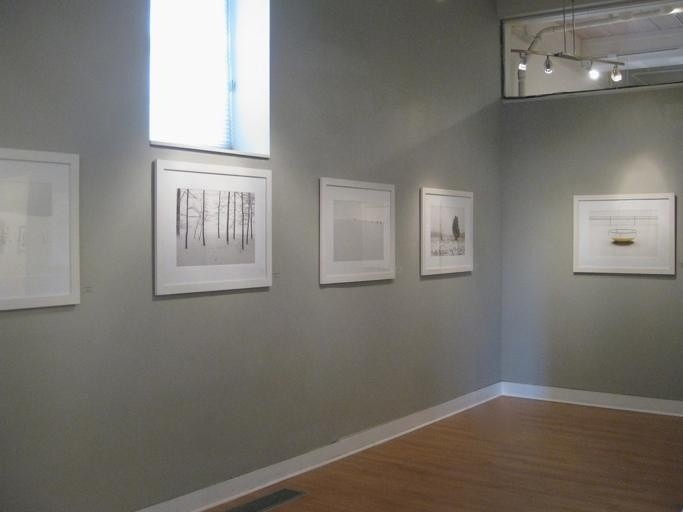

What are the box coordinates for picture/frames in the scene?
[319,173,397,284]
[0,146,82,310]
[155,157,275,296]
[418,184,475,277]
[570,190,677,278]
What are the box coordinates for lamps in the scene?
[510,1,626,84]
